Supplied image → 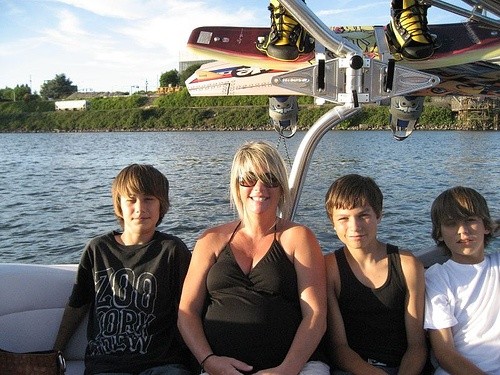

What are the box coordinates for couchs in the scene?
[0,263,91,375]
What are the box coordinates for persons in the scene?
[54,164,200,375]
[423,185,500,375]
[176,139,327,375]
[321,174,426,375]
[261,0,439,62]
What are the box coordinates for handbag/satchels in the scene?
[0,348,66,375]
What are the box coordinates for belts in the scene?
[364,356,399,368]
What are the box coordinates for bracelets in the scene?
[200,354,216,370]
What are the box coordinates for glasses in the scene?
[236,171,281,188]
